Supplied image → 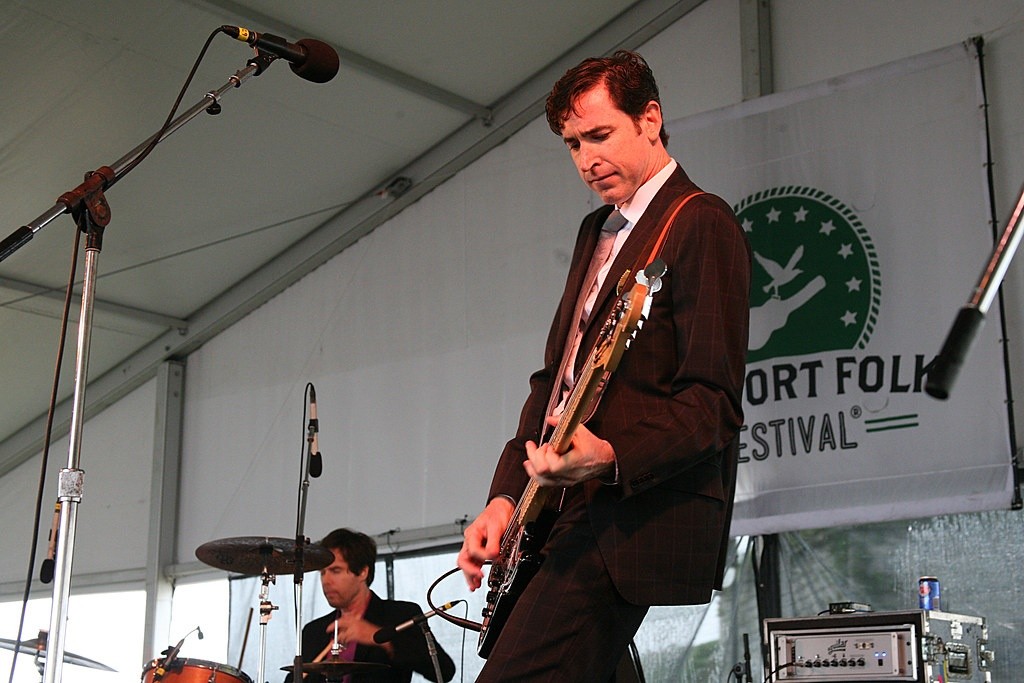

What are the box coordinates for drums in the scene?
[141,657,253,683]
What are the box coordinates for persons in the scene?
[284,528,455,683]
[456,48,752,683]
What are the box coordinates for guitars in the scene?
[478,272,646,657]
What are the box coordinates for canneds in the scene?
[918,576,941,610]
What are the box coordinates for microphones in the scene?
[373,600,460,644]
[154,627,203,681]
[309,384,323,478]
[39,500,62,584]
[221,24,340,84]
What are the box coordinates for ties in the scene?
[540,210,629,449]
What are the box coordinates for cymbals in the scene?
[1,636,117,671]
[280,661,386,675]
[196,537,335,575]
